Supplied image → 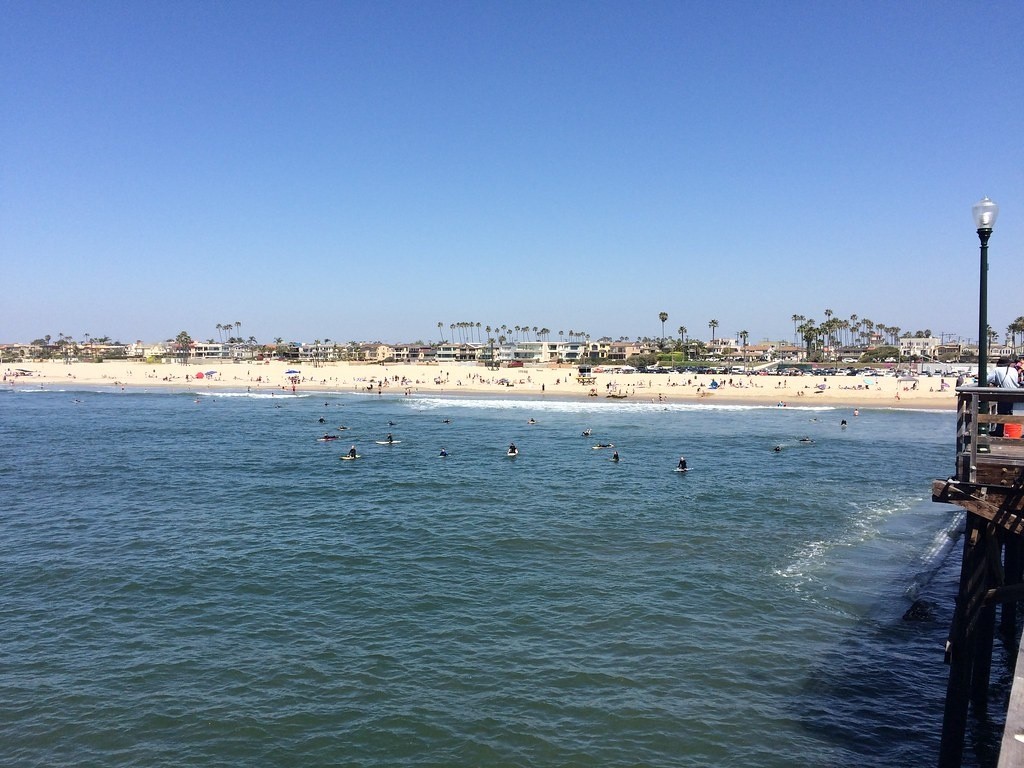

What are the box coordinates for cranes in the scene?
[938,332,957,345]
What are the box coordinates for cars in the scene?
[885,357,896,363]
[509,360,523,367]
[946,359,957,363]
[578,365,972,378]
[842,357,858,363]
[915,358,926,363]
[254,355,265,360]
[694,357,767,362]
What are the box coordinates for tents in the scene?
[897,376,919,389]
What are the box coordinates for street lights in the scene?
[973,198,999,454]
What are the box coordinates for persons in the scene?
[987,353,1024,437]
[316,416,847,471]
[3,368,935,407]
[854,408,858,415]
